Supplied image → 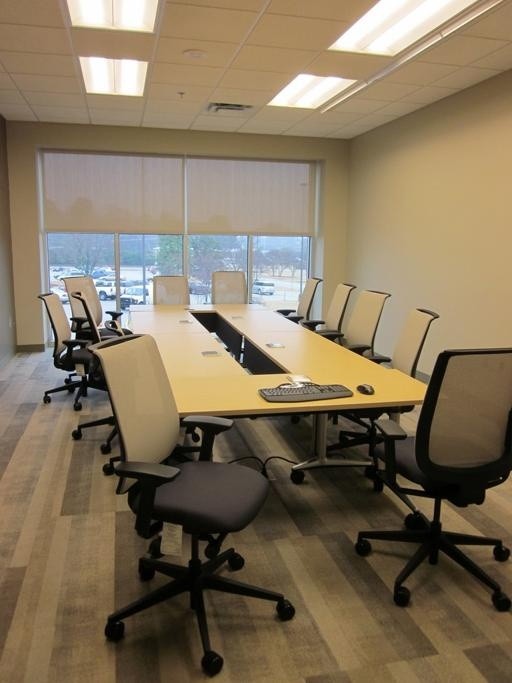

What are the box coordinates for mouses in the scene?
[356,384,374,395]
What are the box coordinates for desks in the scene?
[128,303,429,472]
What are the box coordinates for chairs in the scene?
[88,333,294,675]
[354,348,511,613]
[153,275,191,306]
[303,283,356,343]
[276,276,323,323]
[212,270,247,305]
[333,288,391,430]
[328,305,439,489]
[60,277,133,376]
[37,291,108,409]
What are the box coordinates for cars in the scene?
[50,286,72,304]
[188,280,210,295]
[53,262,126,282]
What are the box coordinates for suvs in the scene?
[117,284,152,311]
[253,280,276,296]
[92,279,118,301]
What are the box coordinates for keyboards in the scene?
[257,382,354,402]
